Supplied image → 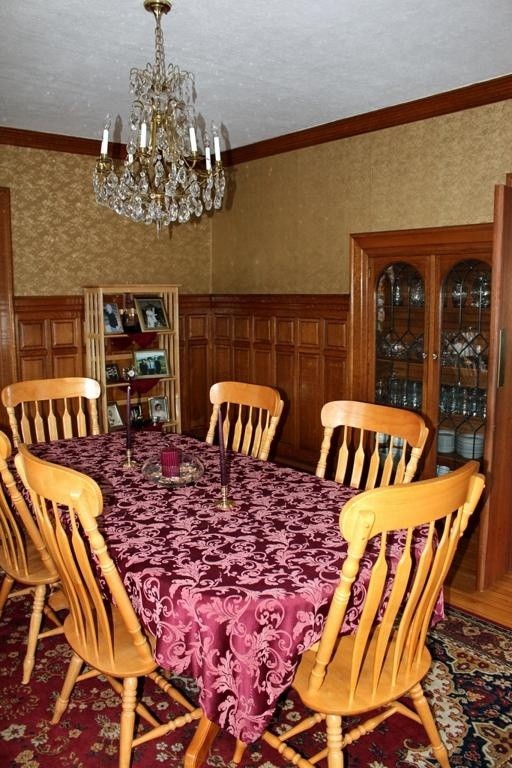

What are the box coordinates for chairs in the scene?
[0,377,101,447]
[260,459,485,768]
[207,381,285,460]
[316,399,429,492]
[0,431,61,684]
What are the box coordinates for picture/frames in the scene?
[103,298,170,430]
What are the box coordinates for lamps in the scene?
[93,0,225,228]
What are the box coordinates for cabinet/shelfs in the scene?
[83,283,183,433]
[350,223,512,594]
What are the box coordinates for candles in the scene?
[218,406,227,486]
[126,383,132,449]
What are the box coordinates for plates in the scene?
[139,453,206,488]
[372,422,485,477]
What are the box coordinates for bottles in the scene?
[376,377,487,417]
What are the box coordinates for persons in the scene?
[139,355,161,375]
[152,403,166,417]
[108,409,115,425]
[103,303,119,332]
[145,304,163,328]
